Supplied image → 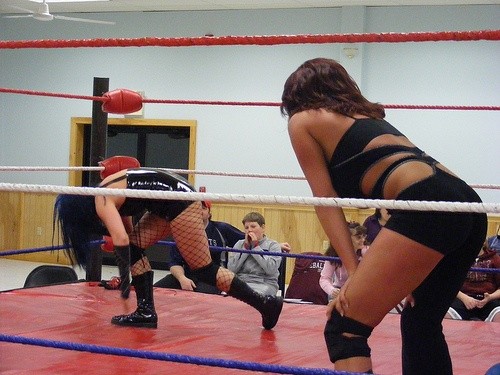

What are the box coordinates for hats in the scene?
[201,199,212,210]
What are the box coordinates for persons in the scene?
[151,201,499,322]
[279,58,488,375]
[51,166,283,330]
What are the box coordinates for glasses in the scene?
[351,235,368,243]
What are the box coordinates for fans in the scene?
[3,0,115,25]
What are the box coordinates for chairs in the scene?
[24,265,78,289]
[277,249,287,300]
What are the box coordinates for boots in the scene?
[110,270,159,328]
[226,275,284,330]
